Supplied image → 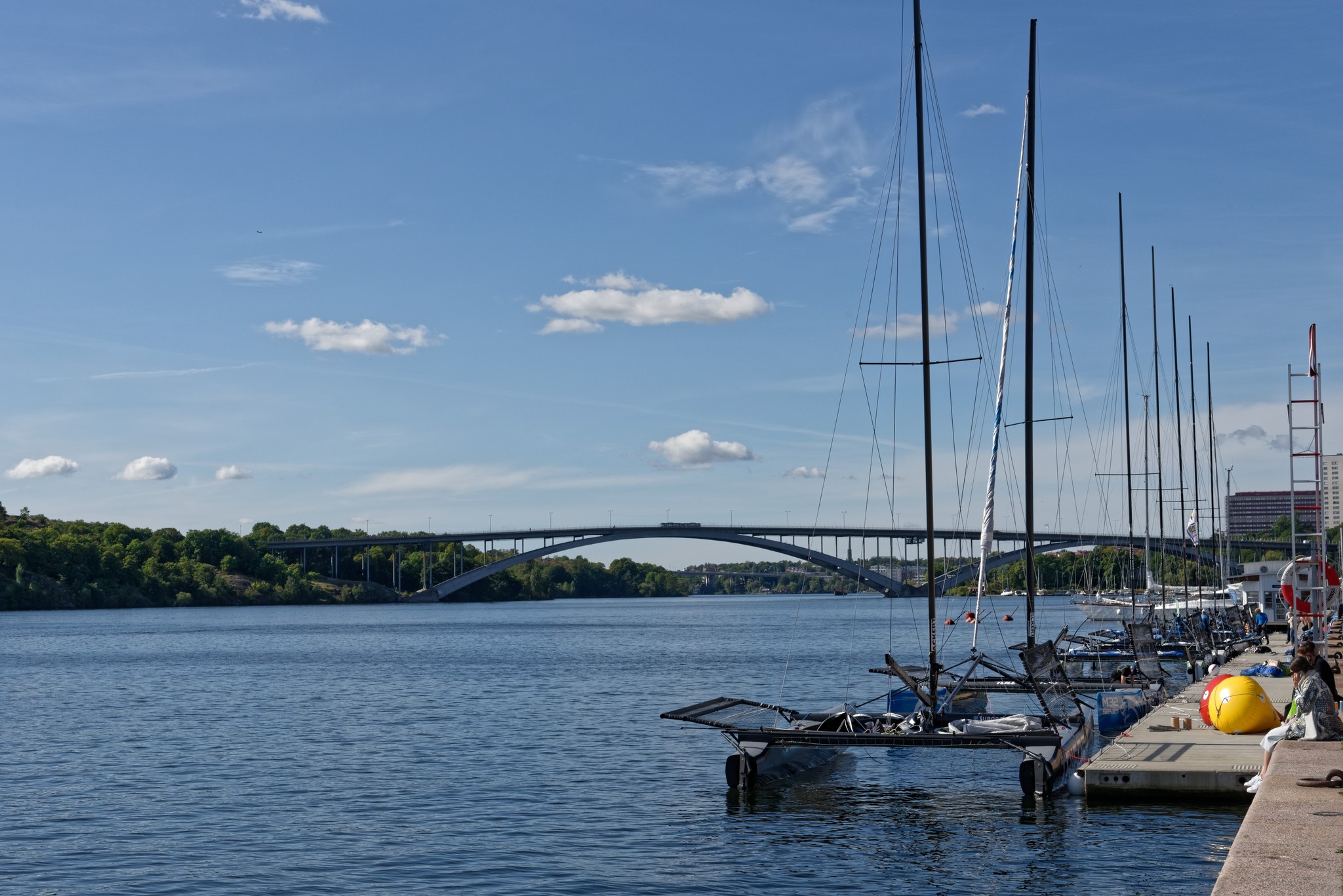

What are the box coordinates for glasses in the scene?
[1257,611,1259,613]
[1289,671,1297,677]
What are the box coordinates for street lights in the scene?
[785,510,790,528]
[428,518,431,533]
[665,509,670,525]
[549,512,553,529]
[729,510,734,527]
[896,513,901,530]
[1004,516,1009,532]
[841,511,846,529]
[303,522,306,539]
[366,520,369,535]
[608,510,613,528]
[239,525,242,537]
[1271,526,1278,541]
[950,514,956,531]
[489,515,492,532]
[1113,519,1119,536]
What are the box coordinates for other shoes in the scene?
[1266,641,1269,645]
[1259,643,1262,646]
[1286,640,1290,644]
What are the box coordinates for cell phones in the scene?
[1305,655,1312,664]
[1296,670,1305,681]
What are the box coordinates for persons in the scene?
[1239,629,1261,647]
[1285,606,1294,644]
[1110,664,1138,684]
[1197,610,1211,638]
[1255,608,1269,645]
[1284,640,1343,721]
[1244,657,1343,793]
[1288,612,1300,647]
[1298,612,1313,632]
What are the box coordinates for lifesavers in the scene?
[1281,557,1340,613]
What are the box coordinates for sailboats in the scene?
[661,0,1262,810]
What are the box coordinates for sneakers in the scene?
[1247,777,1263,793]
[1290,644,1293,647]
[1244,771,1261,788]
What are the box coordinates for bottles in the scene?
[1335,702,1338,712]
[1340,701,1343,712]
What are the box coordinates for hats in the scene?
[1202,609,1206,612]
[1132,664,1138,676]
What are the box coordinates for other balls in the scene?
[1208,664,1220,676]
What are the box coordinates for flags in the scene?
[1228,588,1237,606]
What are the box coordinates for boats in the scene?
[834,591,848,596]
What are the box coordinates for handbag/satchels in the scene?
[1267,657,1281,667]
